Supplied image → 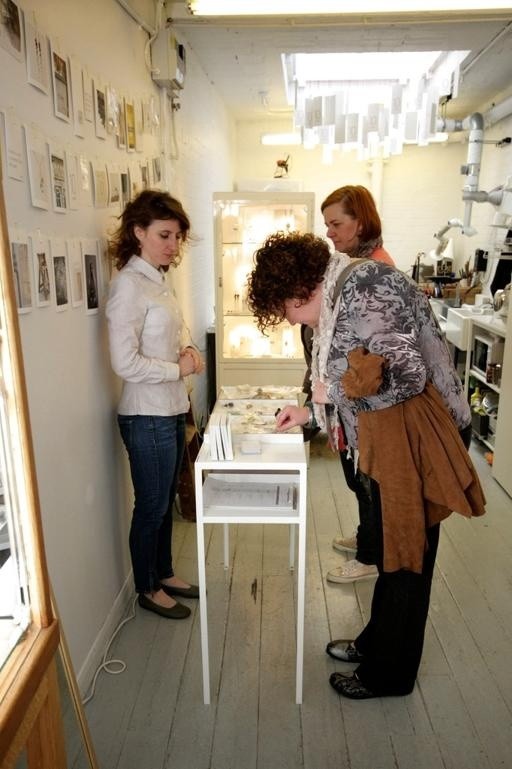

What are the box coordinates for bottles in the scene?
[486,362,502,383]
[470,388,482,407]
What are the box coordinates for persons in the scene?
[246,228,486,701]
[318,184,394,585]
[104,191,209,622]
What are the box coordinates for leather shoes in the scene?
[162,583,208,599]
[139,594,191,620]
[326,639,364,663]
[329,671,381,699]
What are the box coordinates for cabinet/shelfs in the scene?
[1,196,96,766]
[213,195,313,382]
[465,315,511,501]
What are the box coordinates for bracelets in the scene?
[180,350,198,374]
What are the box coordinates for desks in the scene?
[193,385,308,707]
[427,295,485,368]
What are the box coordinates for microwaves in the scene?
[472,336,503,380]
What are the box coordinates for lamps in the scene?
[290,80,436,170]
[177,2,512,24]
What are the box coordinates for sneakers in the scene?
[333,531,357,553]
[326,559,379,584]
